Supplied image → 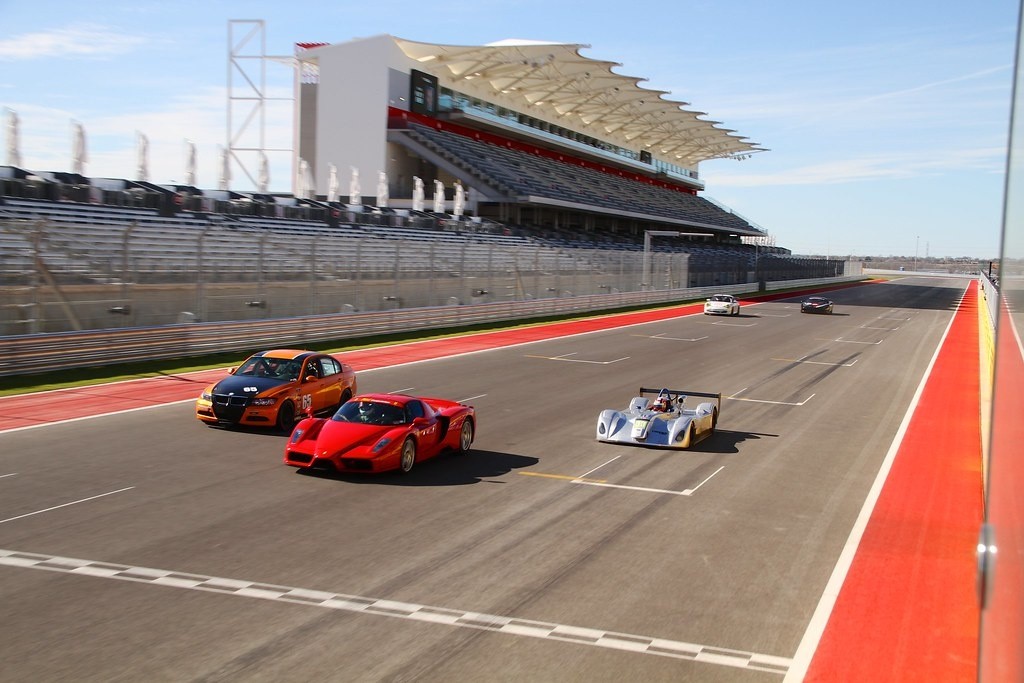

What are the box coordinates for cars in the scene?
[703,294,741,317]
[193,348,357,433]
[800,296,835,315]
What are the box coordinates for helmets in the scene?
[290,363,302,376]
[654,397,671,412]
[360,402,375,415]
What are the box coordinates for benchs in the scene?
[407,123,760,231]
[0,196,844,322]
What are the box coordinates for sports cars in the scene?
[281,389,476,481]
[595,387,723,449]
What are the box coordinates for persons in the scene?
[353,403,378,424]
[652,397,672,414]
